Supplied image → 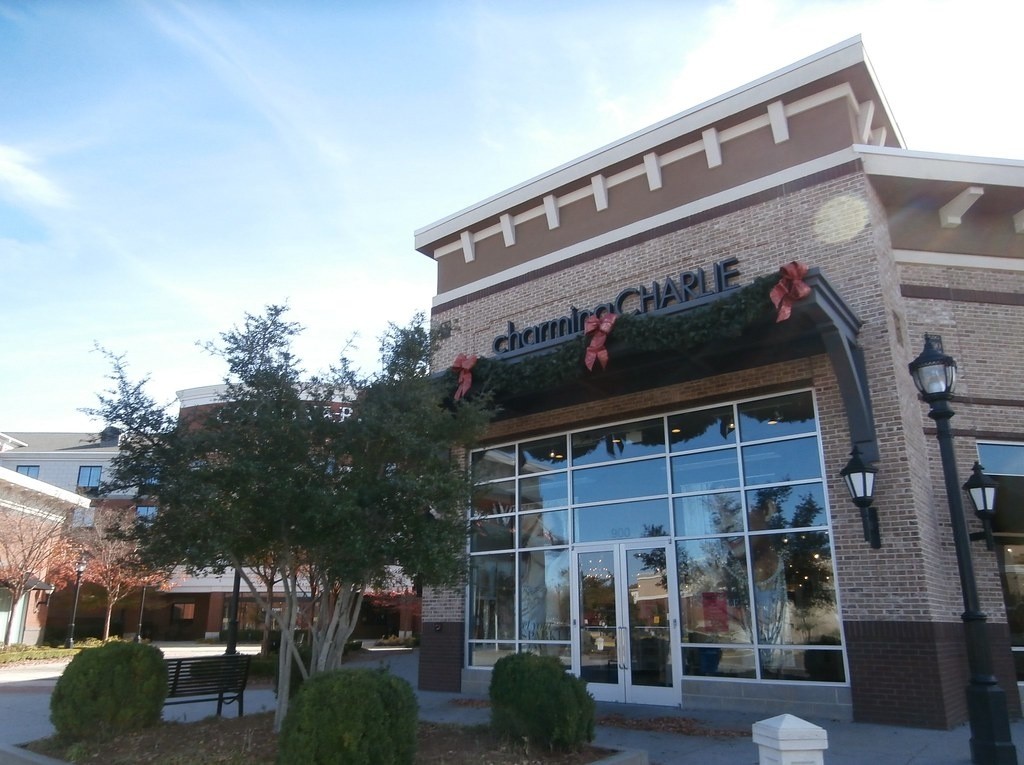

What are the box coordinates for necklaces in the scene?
[722,562,784,585]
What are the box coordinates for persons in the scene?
[708,478,817,669]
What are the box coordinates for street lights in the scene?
[907,331,1019,765]
[132,568,151,643]
[63,555,87,649]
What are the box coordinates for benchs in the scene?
[155,656,252,719]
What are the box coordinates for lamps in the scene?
[839,446,883,550]
[962,463,998,551]
[550,408,783,458]
